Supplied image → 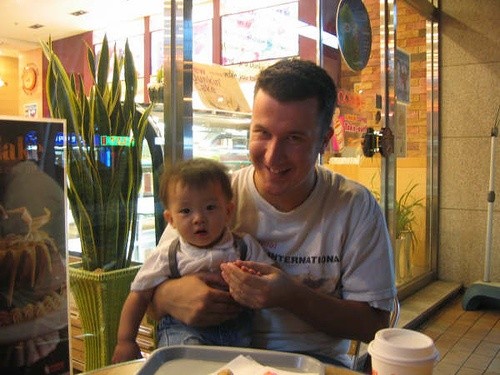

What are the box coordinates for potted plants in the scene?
[37,31,154,372]
[395,185,425,278]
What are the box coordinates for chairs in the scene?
[346,293,400,357]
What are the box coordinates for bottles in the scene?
[368,327,440,372]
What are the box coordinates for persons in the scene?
[145,58,398,371]
[110,157,284,364]
